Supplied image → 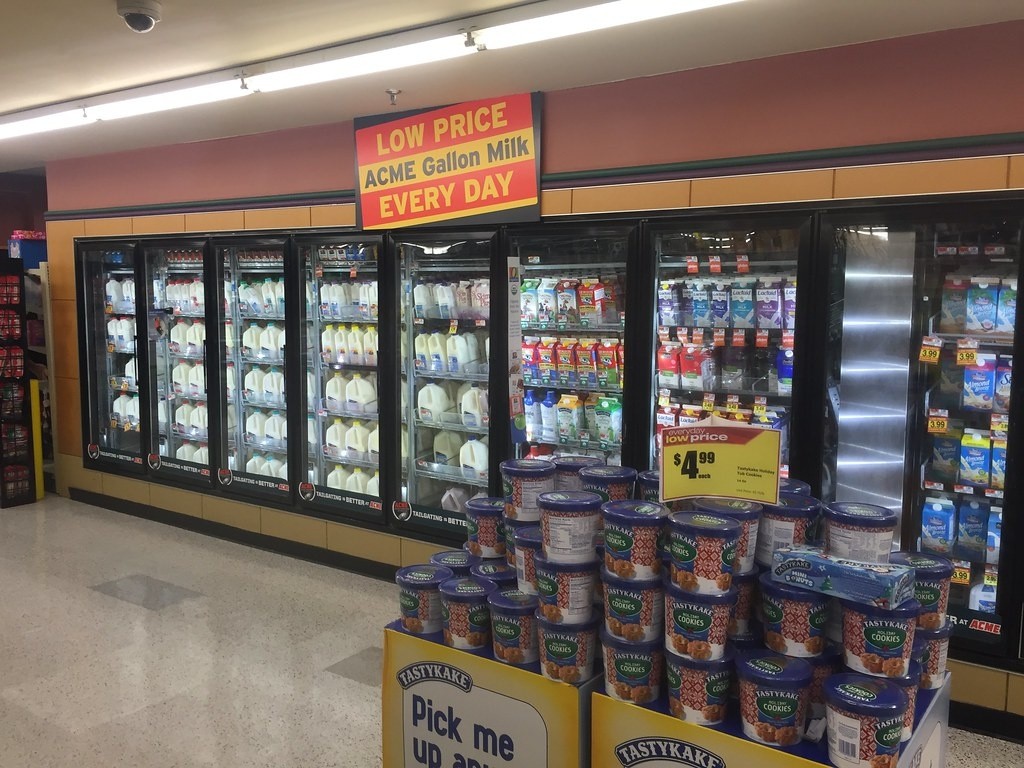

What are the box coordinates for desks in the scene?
[384,618,951,768]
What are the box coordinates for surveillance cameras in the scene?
[116,0,162,34]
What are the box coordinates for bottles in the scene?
[97,249,167,455]
[415,276,490,515]
[537,389,558,443]
[524,387,540,442]
[320,241,378,496]
[241,244,318,485]
[168,244,238,469]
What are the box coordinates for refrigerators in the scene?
[639,215,916,555]
[71,224,634,546]
[900,218,1023,671]
